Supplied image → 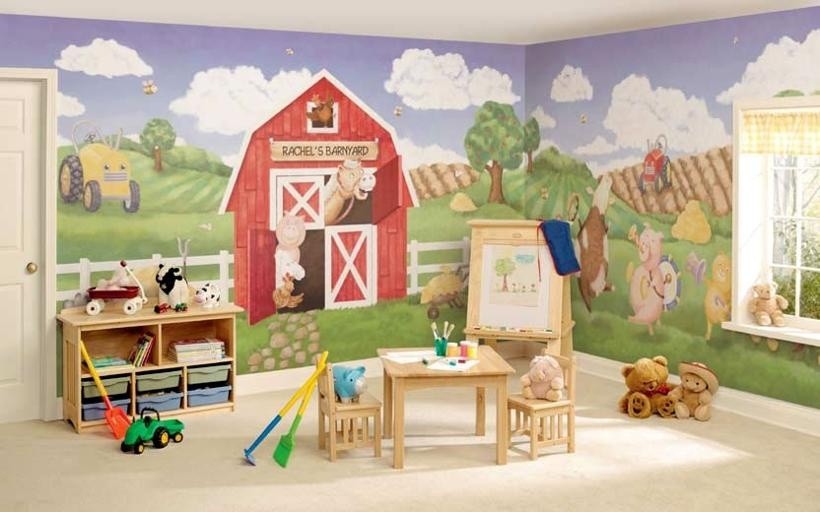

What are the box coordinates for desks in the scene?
[374,345,517,469]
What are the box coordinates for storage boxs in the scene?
[81,363,233,421]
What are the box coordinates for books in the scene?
[82,331,227,371]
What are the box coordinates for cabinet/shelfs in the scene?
[59,303,246,435]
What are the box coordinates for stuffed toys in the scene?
[617,355,677,419]
[520,355,564,402]
[196,281,223,308]
[155,264,190,309]
[666,362,719,422]
[333,364,366,405]
[747,282,788,327]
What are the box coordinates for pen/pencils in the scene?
[431,321,454,339]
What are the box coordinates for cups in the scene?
[467,342,477,358]
[447,342,458,356]
[460,340,470,356]
[435,339,446,356]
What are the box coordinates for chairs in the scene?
[315,360,384,464]
[505,350,576,460]
